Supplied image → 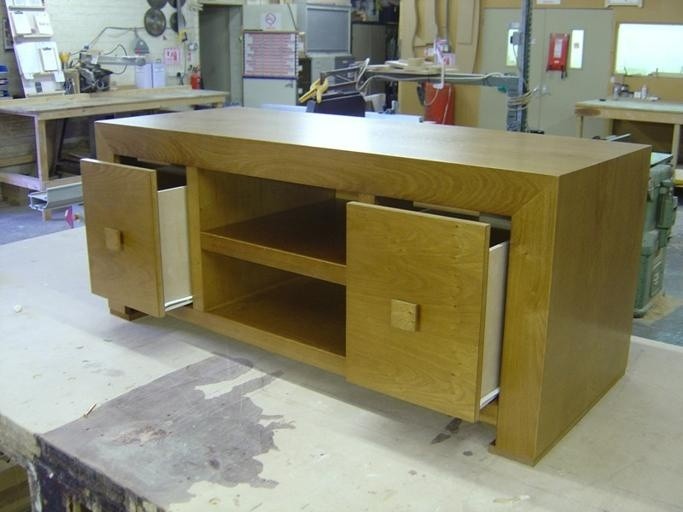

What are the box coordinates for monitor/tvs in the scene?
[612,20,683,78]
[303,4,353,56]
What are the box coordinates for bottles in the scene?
[613,84,634,98]
[642,84,648,100]
[0,64,9,97]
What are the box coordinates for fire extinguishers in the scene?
[191,66,202,89]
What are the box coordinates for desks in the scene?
[81,107,651,468]
[0,85,230,223]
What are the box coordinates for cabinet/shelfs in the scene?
[574,99,683,169]
[346,194,513,424]
[193,167,356,358]
[80,152,193,320]
